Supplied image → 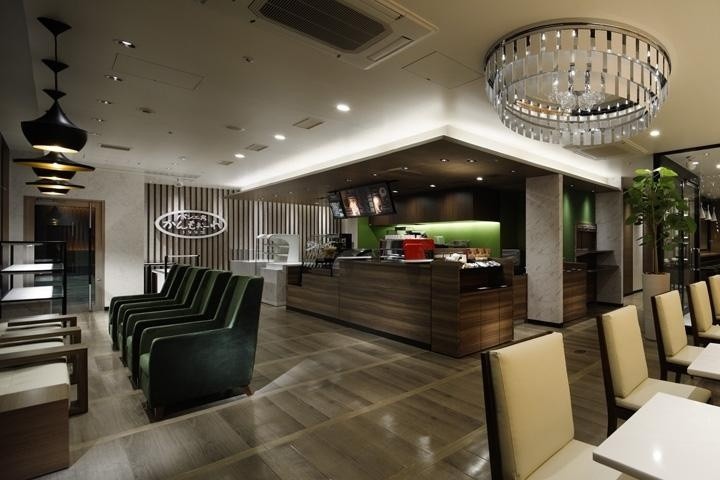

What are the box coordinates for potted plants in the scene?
[624,166,695,343]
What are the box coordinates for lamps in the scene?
[698,174,717,221]
[482,17,672,146]
[25,179,85,189]
[13,151,96,172]
[19,15,87,153]
[31,166,75,181]
[37,187,71,196]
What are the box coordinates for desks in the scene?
[591,391,719,480]
[686,343,720,381]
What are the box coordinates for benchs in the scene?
[0,313,89,480]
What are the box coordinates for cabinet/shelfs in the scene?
[0,240,67,338]
[229,233,300,308]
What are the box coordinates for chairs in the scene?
[649,288,705,384]
[109,264,264,423]
[687,280,720,348]
[706,275,720,324]
[481,330,636,480]
[595,303,712,437]
